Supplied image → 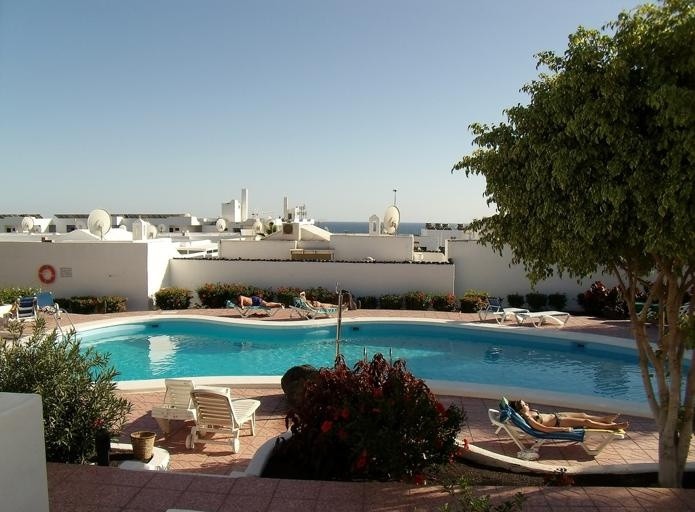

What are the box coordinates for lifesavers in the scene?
[38,265,55,283]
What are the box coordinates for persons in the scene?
[299,291,312,305]
[511,399,629,434]
[234,294,284,309]
[311,299,346,308]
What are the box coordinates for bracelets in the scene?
[563,427,569,432]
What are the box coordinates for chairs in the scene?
[34,292,63,315]
[10,296,39,321]
[288,297,338,320]
[487,397,626,461]
[151,378,232,434]
[227,300,282,319]
[516,311,570,328]
[185,388,261,454]
[478,297,503,323]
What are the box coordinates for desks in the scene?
[493,307,530,327]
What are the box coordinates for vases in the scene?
[129,430,157,460]
